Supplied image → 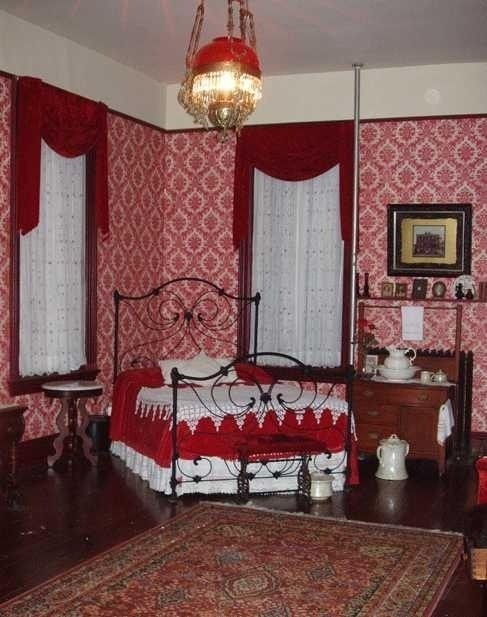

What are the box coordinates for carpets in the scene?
[0,501,465,617]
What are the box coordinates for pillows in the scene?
[157,356,239,387]
[126,363,273,387]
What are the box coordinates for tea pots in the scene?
[431,369,449,384]
[384,345,417,370]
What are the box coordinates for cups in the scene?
[309,472,335,502]
[420,371,435,385]
[372,432,412,482]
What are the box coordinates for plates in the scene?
[378,367,422,382]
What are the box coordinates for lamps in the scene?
[178,0,261,138]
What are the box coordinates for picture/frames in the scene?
[387,202,472,275]
[381,282,393,297]
[396,282,407,299]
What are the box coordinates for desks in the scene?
[41,380,103,477]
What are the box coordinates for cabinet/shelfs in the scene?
[351,302,462,479]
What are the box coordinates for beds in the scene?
[111,278,353,507]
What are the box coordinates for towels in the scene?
[400,306,424,340]
[438,399,455,446]
